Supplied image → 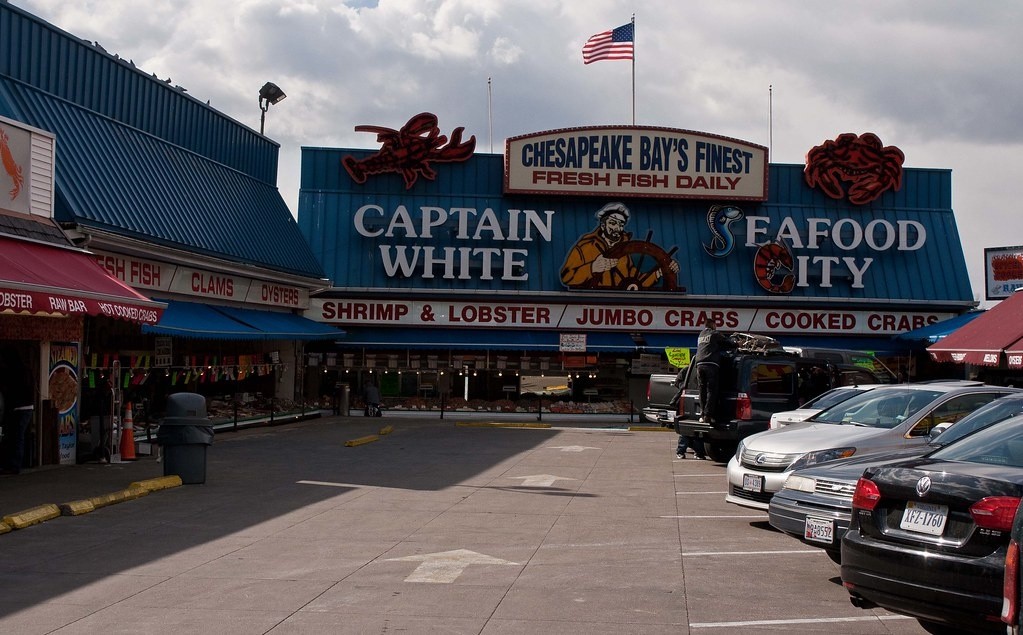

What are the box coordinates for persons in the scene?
[670,366,707,460]
[363,380,382,417]
[695,318,744,422]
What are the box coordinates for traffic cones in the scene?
[121,402,138,460]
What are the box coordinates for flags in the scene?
[582,23,633,65]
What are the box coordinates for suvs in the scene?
[674,351,844,461]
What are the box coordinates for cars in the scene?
[768,383,904,430]
[1001,496,1023,635]
[725,381,1023,511]
[841,412,1023,635]
[769,392,1023,566]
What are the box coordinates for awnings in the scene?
[926,286,1023,369]
[333,326,896,355]
[0,236,169,325]
[899,309,989,345]
[139,298,347,342]
[1004,338,1023,369]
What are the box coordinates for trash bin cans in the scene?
[157,391,213,485]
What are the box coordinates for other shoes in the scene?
[0,468,17,477]
[677,454,685,459]
[694,456,706,460]
[699,415,711,423]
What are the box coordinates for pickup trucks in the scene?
[642,346,909,446]
